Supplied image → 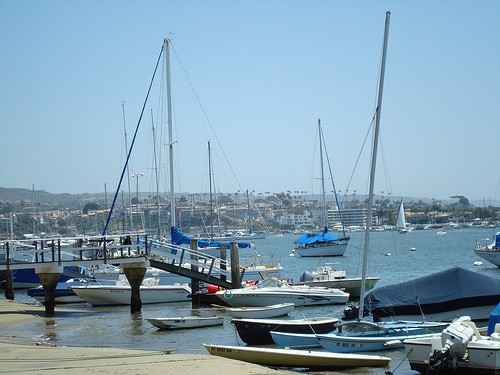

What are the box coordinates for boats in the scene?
[201,342,392,369]
[472,231,500,268]
[400,316,500,375]
[144,317,225,330]
[209,302,295,319]
[70,273,193,306]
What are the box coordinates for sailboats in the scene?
[0,11,500,355]
[395,199,410,234]
[289,119,351,258]
